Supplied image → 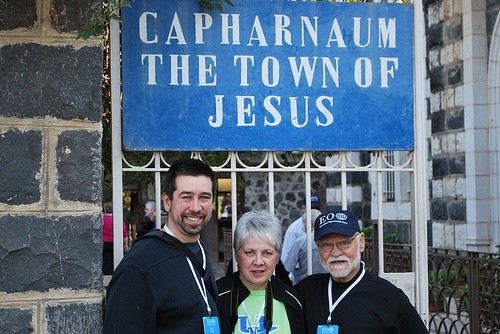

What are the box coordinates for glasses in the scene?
[316,233,360,250]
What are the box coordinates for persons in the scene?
[143,201,168,235]
[293,210,427,334]
[102,158,224,334]
[280,192,335,283]
[102,202,137,275]
[215,209,305,334]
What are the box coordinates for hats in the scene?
[313,210,361,242]
